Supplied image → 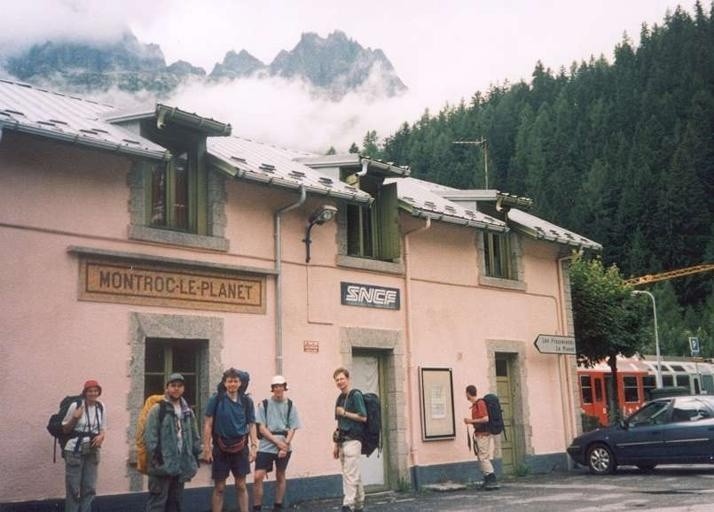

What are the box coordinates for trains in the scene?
[576,357,714,428]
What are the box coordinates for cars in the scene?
[565,394,714,475]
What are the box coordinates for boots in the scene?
[476,473,501,492]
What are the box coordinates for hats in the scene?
[166,373,185,387]
[82,379,102,396]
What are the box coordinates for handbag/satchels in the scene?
[332,429,346,443]
[215,435,249,455]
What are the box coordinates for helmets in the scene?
[270,375,289,392]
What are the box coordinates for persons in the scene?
[202,369,260,511]
[143,372,201,512]
[255,372,300,511]
[59,379,108,512]
[463,383,499,491]
[333,368,368,511]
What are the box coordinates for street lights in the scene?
[631,288,663,388]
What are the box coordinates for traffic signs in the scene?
[533,333,578,354]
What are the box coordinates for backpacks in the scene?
[336,389,381,458]
[211,368,250,436]
[473,394,504,435]
[45,396,104,449]
[134,393,189,475]
[255,398,293,440]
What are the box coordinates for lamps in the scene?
[306,205,338,263]
